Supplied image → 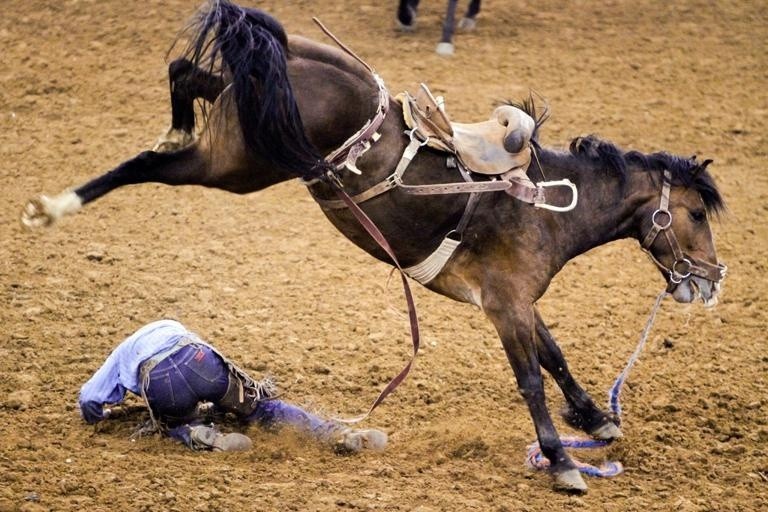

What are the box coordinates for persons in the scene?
[79,320,389,452]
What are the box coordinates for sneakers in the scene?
[189,424,253,454]
[329,426,390,456]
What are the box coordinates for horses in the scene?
[19,0,727,495]
[393,0,481,55]
